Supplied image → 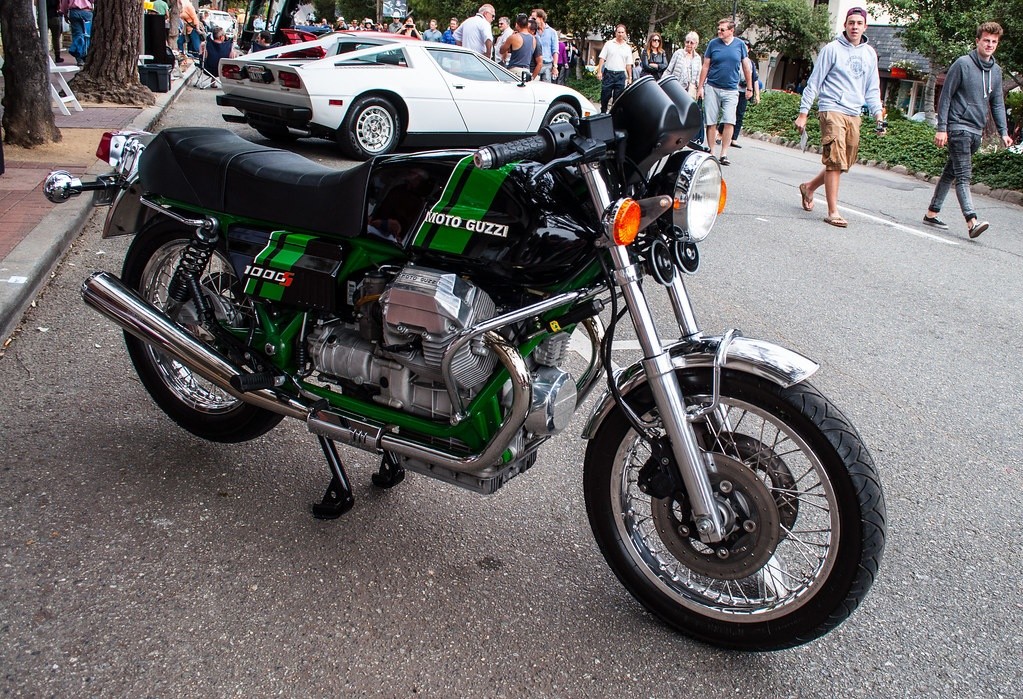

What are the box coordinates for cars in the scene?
[1007,141,1023,156]
[213,25,596,162]
[908,112,940,125]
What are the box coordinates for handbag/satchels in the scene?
[749,75,757,103]
[695,95,704,144]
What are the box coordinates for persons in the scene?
[922,22,1013,239]
[640,33,668,81]
[36,0,581,88]
[661,31,704,145]
[794,7,886,227]
[696,19,753,164]
[589,56,595,66]
[597,24,634,113]
[715,37,760,148]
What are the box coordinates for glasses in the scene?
[487,12,495,18]
[717,28,727,32]
[651,39,661,42]
[685,41,695,45]
[393,18,399,20]
[498,23,504,25]
[532,18,537,21]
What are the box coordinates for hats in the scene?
[363,19,375,26]
[391,11,404,18]
[351,20,359,24]
[565,33,574,40]
[337,16,345,22]
[844,7,868,21]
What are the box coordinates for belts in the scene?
[606,71,625,75]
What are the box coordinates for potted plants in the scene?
[899,103,905,112]
[887,60,929,80]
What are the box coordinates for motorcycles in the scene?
[40,62,888,653]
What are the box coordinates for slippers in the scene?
[823,216,848,226]
[800,183,817,210]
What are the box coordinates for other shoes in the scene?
[730,142,743,148]
[56,55,65,63]
[77,59,86,66]
[720,156,733,166]
[716,139,724,145]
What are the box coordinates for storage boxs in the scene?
[138,64,172,93]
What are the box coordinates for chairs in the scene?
[193,35,233,90]
[138,54,154,78]
[35,32,84,115]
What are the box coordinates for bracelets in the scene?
[747,89,753,92]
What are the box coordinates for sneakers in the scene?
[923,214,950,229]
[968,218,989,238]
